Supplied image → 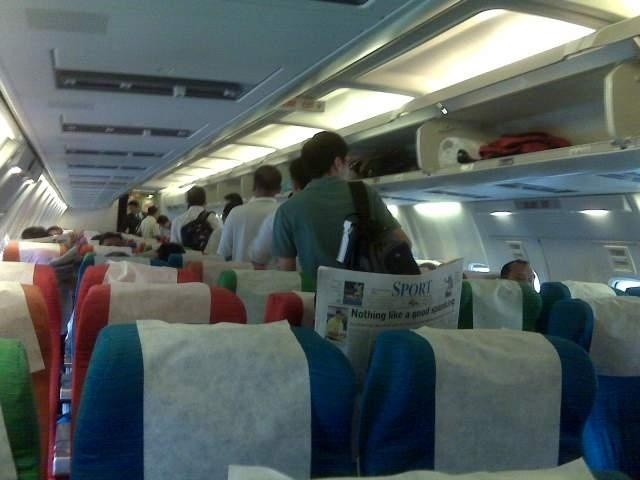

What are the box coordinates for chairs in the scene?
[0,281,53,480]
[53,229,76,250]
[70,319,359,480]
[548,298,640,480]
[265,291,317,326]
[79,230,103,245]
[0,261,61,480]
[178,261,256,283]
[168,254,227,269]
[122,232,202,266]
[355,326,631,480]
[78,252,175,300]
[75,265,201,335]
[3,241,68,265]
[540,281,630,304]
[71,282,248,456]
[81,245,140,256]
[0,339,41,479]
[214,268,315,324]
[458,278,543,332]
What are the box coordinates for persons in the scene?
[271,131,413,292]
[100,231,124,246]
[169,186,223,252]
[202,201,242,255]
[248,158,312,271]
[216,165,282,265]
[118,199,142,237]
[500,259,535,287]
[137,205,161,241]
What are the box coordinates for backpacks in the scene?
[335,181,421,275]
[478,132,572,159]
[350,146,418,177]
[181,210,216,250]
